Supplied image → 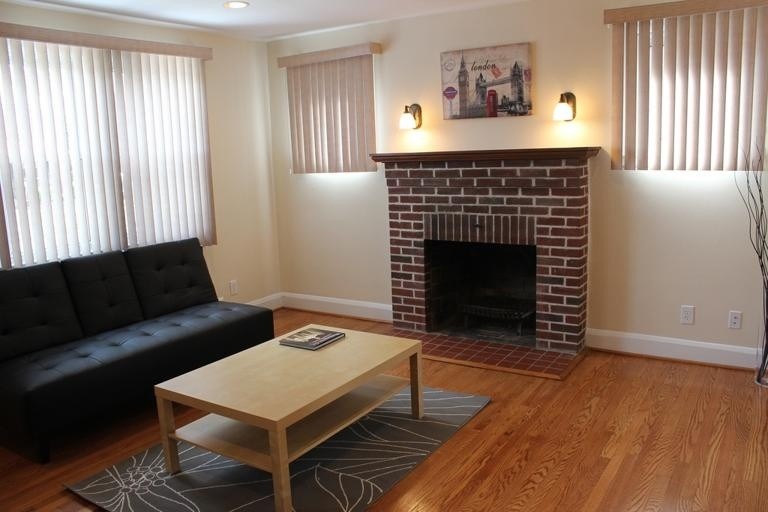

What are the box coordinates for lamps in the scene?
[399,103,423,129]
[552,91,576,122]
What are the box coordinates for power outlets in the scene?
[726,310,743,329]
[679,305,696,325]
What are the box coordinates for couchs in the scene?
[1,235,275,468]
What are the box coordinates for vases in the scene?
[753,275,767,388]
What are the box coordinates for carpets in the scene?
[61,382,491,512]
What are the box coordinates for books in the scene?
[279,327,345,351]
